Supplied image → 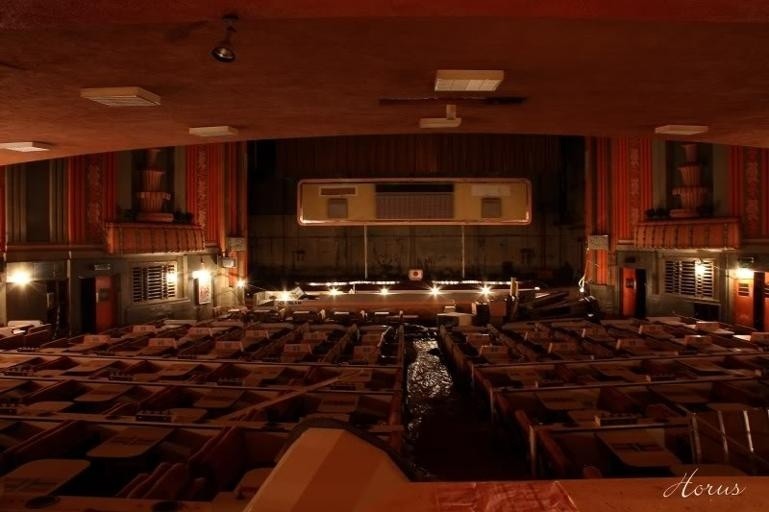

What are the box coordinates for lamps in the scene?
[210,24,236,63]
